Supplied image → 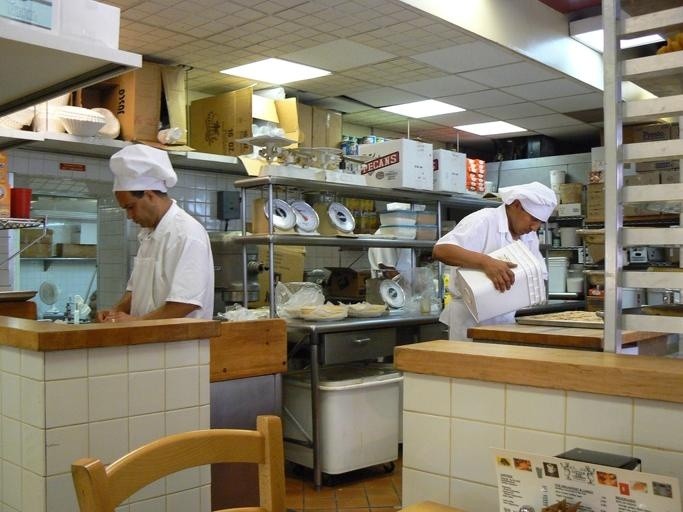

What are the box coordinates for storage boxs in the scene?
[559,121,683,223]
[95,65,341,168]
[357,139,467,195]
[325,264,400,302]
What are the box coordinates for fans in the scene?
[39,281,62,314]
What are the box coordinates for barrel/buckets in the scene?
[455,242,546,323]
[621,286,672,309]
[559,183,583,203]
[548,256,570,293]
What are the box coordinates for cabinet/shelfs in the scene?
[238,177,506,490]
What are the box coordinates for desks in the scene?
[468,323,669,358]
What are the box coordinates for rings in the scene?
[112,319,115,323]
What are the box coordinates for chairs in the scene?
[71,415,292,512]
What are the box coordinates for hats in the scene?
[498,181,558,223]
[107,143,177,194]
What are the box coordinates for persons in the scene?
[96,144,214,323]
[433,182,558,342]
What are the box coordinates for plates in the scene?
[283,301,386,322]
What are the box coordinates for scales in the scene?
[251,185,355,236]
[360,268,405,308]
